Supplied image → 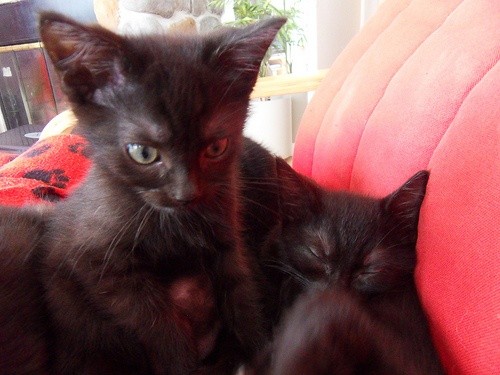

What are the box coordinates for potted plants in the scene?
[206,0,306,161]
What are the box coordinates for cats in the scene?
[232,155,451,375]
[0,8,282,375]
